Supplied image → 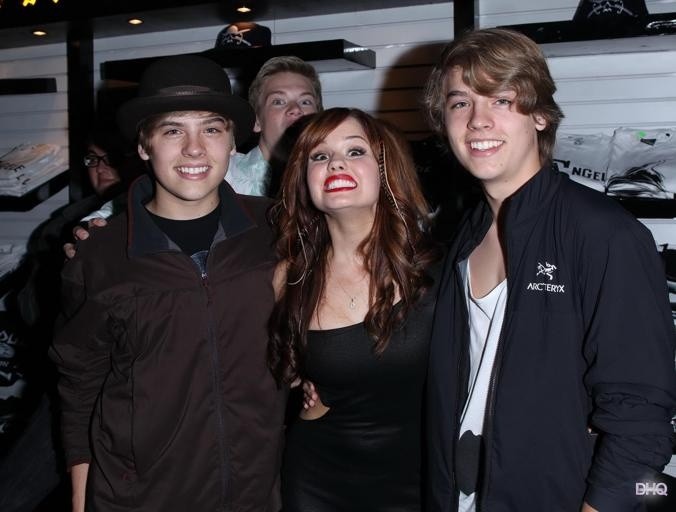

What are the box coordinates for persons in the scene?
[50,58,298,511]
[274,106,447,511]
[0,127,122,511]
[292,24,675,512]
[63,54,324,258]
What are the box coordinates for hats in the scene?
[130,54,256,143]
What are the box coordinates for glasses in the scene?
[81,153,116,168]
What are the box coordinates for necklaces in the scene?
[329,256,373,309]
